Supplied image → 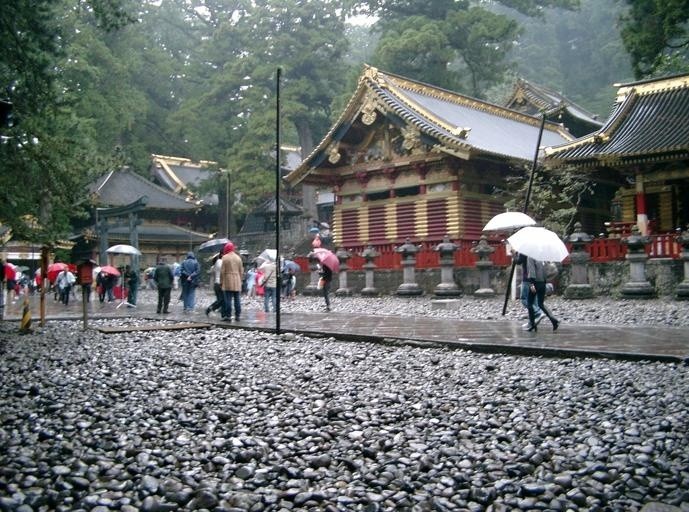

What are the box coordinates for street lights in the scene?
[217,166,233,238]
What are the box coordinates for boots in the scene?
[550,315,560,331]
[526,319,538,333]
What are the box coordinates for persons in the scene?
[1,260,77,306]
[524,256,560,331]
[83,241,276,322]
[283,262,332,312]
[511,252,546,328]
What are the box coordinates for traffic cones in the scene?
[13,297,38,336]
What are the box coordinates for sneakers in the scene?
[156,310,170,314]
[534,314,546,325]
[234,314,240,323]
[220,316,233,323]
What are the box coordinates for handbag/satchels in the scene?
[543,260,559,282]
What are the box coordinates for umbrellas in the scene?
[507,226,570,263]
[482,208,537,235]
[313,247,340,274]
[310,227,320,232]
[321,222,331,229]
